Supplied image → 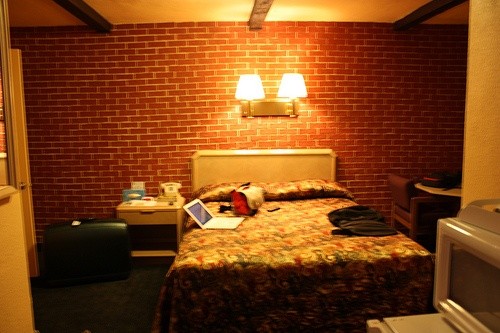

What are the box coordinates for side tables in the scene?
[117,195,186,258]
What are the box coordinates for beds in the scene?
[152,149,433,333]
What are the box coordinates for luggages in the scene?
[43,218,131,287]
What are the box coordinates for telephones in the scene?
[160,182,182,196]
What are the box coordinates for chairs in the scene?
[387,172,458,241]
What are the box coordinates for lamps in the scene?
[277,73,308,118]
[234,75,265,119]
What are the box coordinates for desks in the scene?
[414,178,461,197]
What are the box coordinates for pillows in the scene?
[189,181,262,202]
[263,180,354,201]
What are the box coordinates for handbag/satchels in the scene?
[232,185,264,215]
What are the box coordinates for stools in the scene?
[45,218,129,287]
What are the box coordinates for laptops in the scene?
[183,198,245,230]
